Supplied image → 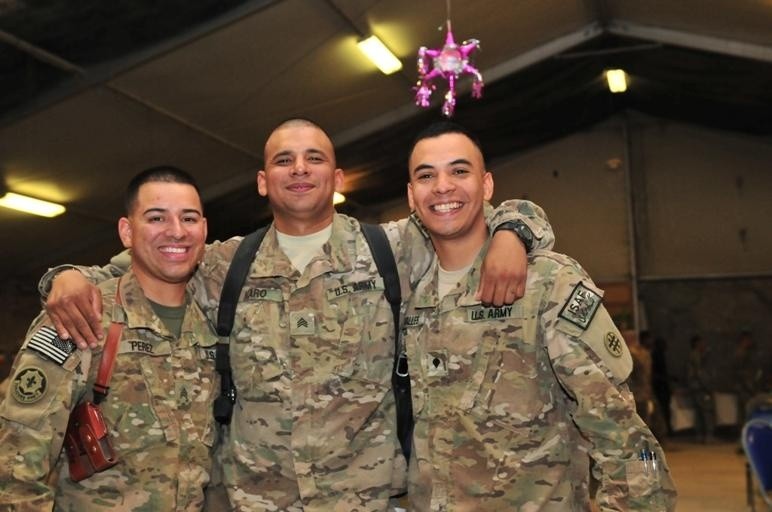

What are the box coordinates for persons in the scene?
[729,332,763,433]
[1,165,230,511]
[37,119,556,509]
[686,337,724,445]
[404,120,676,512]
[640,331,672,438]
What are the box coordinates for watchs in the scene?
[492,222,533,252]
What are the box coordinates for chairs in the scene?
[741,417,771,505]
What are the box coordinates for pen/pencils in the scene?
[641,448,657,475]
